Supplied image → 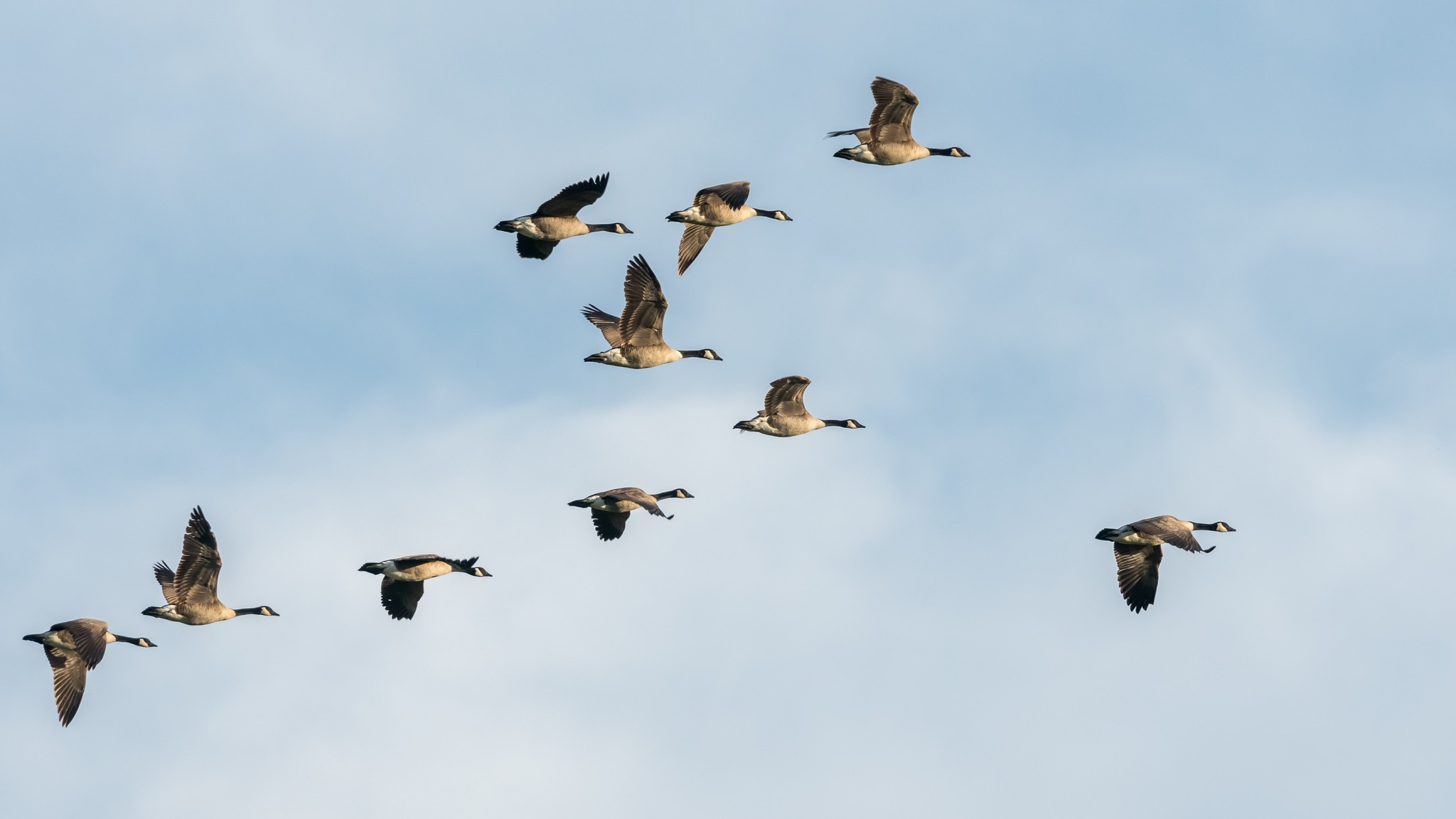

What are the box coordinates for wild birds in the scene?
[494,76,971,437]
[356,554,494,621]
[567,486,695,541]
[21,505,281,725]
[1093,515,1237,614]
